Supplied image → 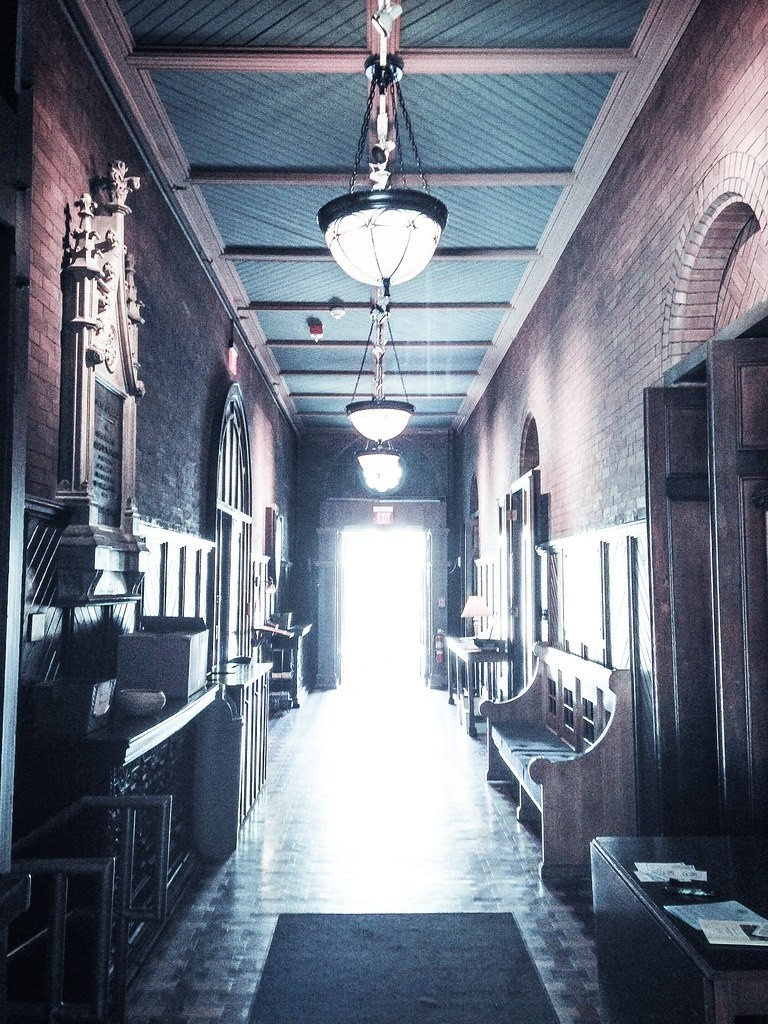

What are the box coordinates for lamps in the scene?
[371,297,391,325]
[316,50,448,297]
[357,439,400,479]
[255,577,278,594]
[345,303,415,445]
[370,1,404,37]
[461,596,492,639]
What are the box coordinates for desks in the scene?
[589,834,768,1024]
[445,636,515,737]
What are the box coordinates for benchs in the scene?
[480,640,633,880]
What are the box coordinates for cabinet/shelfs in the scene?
[197,662,274,850]
[268,622,316,708]
[13,685,215,1003]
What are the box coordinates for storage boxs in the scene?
[270,610,294,629]
[117,615,209,700]
[31,679,118,734]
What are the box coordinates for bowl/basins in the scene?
[470,638,504,652]
[114,688,167,720]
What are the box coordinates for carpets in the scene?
[245,913,560,1024]
